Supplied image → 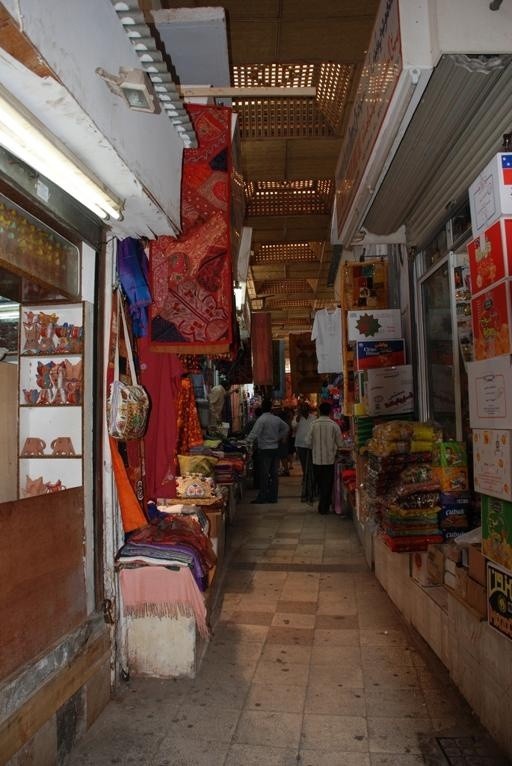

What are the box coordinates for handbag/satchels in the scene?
[108,380,150,440]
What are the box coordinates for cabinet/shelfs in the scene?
[15,297,96,616]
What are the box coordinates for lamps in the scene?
[0,83,128,225]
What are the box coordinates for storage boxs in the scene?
[425,439,468,599]
[424,277,455,411]
[344,306,418,457]
[467,148,512,643]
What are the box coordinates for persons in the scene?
[246,405,298,491]
[305,402,344,515]
[292,402,318,502]
[220,378,241,434]
[245,399,289,503]
